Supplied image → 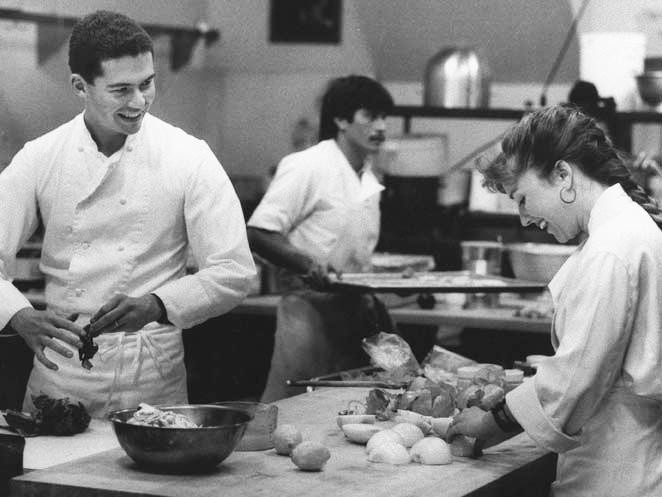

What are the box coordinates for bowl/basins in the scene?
[214,400,276,452]
[634,74,662,104]
[508,243,586,286]
[106,403,253,474]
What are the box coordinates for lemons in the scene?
[290,440,331,469]
[271,423,303,454]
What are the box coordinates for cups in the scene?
[462,238,503,310]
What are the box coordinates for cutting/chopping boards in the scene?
[11,384,560,494]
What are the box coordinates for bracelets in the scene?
[490,402,520,438]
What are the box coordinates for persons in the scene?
[446,102,662,497]
[247,75,392,404]
[0,10,257,420]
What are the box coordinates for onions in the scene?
[335,408,477,464]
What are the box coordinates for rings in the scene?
[113,320,121,328]
